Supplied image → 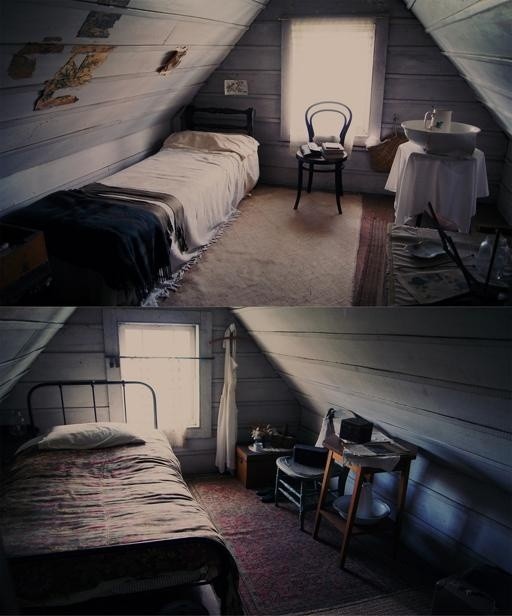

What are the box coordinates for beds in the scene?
[1,380,240,616]
[0,103,262,306]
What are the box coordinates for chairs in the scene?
[273,408,362,531]
[291,100,352,215]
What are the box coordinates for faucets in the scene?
[423,106,442,131]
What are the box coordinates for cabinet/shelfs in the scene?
[236,445,299,490]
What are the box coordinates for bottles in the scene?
[476,236,510,282]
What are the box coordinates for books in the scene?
[300,141,345,160]
[342,441,411,458]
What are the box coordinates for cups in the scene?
[425,107,453,134]
[254,442,263,453]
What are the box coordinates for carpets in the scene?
[160,187,365,306]
[188,474,424,616]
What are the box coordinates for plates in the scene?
[405,239,448,261]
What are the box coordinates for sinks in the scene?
[400,120,482,157]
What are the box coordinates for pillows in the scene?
[159,126,259,159]
[38,420,146,452]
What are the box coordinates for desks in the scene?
[382,222,511,308]
[387,142,488,233]
[312,439,417,572]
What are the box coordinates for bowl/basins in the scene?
[333,495,392,525]
[402,119,483,151]
